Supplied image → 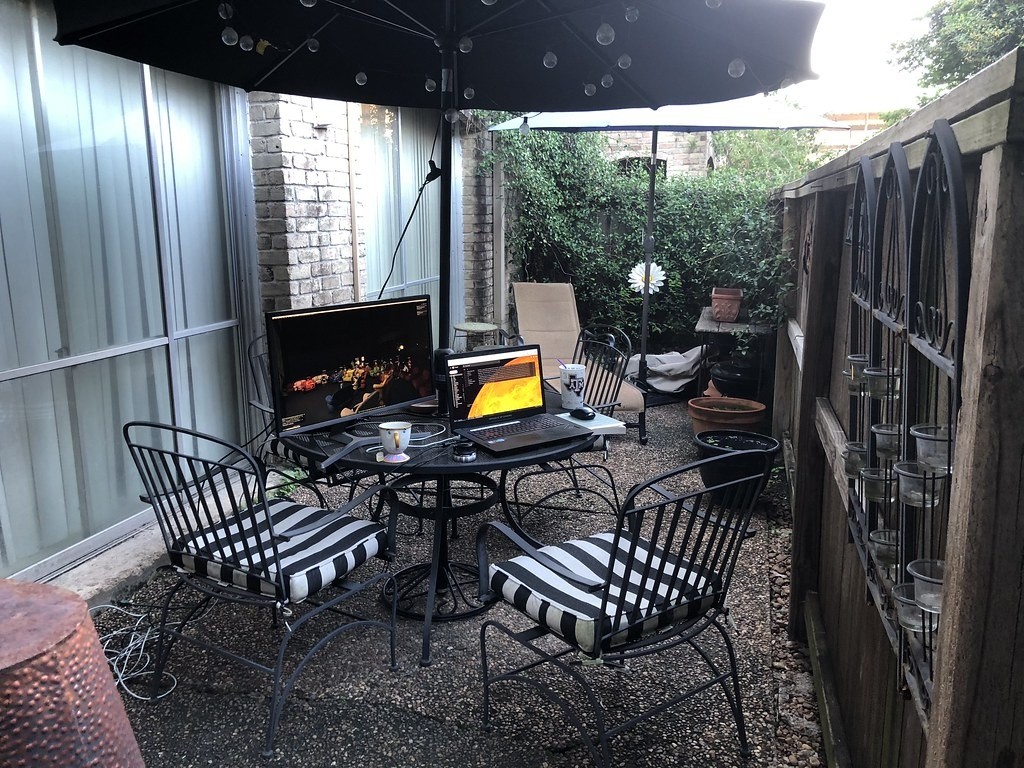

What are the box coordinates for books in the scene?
[554,412,627,435]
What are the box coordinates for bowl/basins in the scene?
[907,559,945,614]
[870,529,901,568]
[845,441,867,479]
[863,367,900,399]
[893,460,946,507]
[860,468,897,503]
[892,583,937,632]
[910,423,958,473]
[847,354,869,383]
[871,423,903,458]
[842,369,861,396]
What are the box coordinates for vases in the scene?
[694,428,781,510]
[688,396,767,437]
[711,287,743,324]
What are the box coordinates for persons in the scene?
[300,354,431,418]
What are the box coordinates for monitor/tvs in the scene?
[264,294,437,469]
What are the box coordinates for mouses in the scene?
[570,406,596,421]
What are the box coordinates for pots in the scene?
[710,356,764,397]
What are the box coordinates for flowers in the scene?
[628,262,666,295]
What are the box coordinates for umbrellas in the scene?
[53,0,826,591]
[488,92,850,445]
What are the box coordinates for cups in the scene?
[378,422,412,463]
[559,363,586,410]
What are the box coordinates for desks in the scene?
[694,306,776,402]
[280,390,600,667]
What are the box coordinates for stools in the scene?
[451,322,498,351]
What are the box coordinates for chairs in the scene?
[475,449,772,768]
[480,324,633,528]
[498,282,649,446]
[123,420,398,759]
[238,333,425,537]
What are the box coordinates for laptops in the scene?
[443,344,594,456]
